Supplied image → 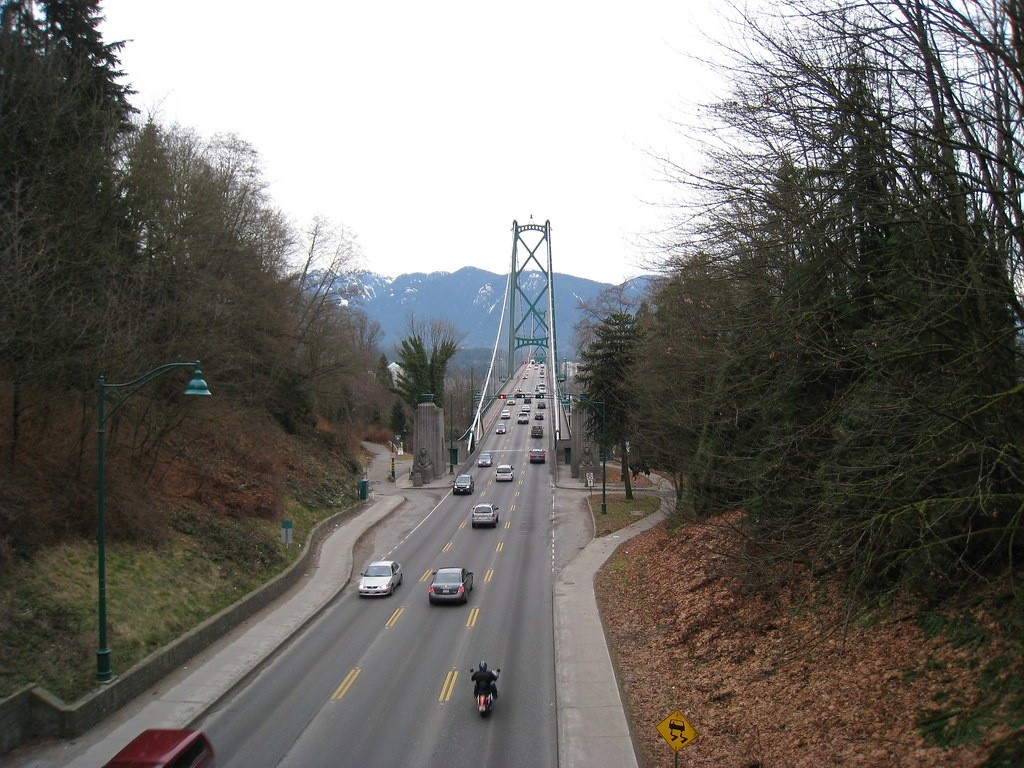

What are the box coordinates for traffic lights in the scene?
[499,395,507,399]
[535,395,544,398]
[517,394,526,398]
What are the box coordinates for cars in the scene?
[477,454,493,467]
[506,359,547,412]
[358,561,403,597]
[501,410,510,420]
[530,425,545,438]
[495,424,507,434]
[428,567,473,606]
[534,412,545,420]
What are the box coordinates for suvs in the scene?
[528,449,546,464]
[496,464,515,482]
[452,473,475,495]
[472,503,499,528]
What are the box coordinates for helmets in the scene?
[479,661,487,670]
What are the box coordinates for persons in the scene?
[471,661,499,699]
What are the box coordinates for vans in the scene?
[102,728,215,768]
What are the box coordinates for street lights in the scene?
[94,360,212,685]
[560,392,607,515]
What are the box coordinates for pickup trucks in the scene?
[516,412,530,424]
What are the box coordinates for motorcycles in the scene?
[469,668,501,719]
